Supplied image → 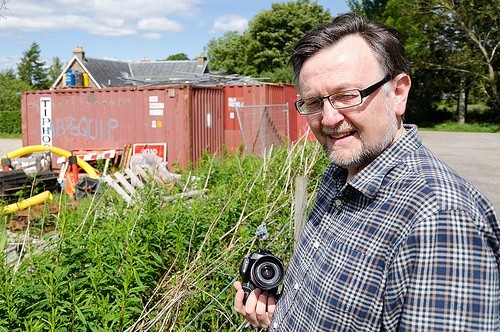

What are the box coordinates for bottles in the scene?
[66,69,74,86]
[75,69,82,86]
[82,73,88,86]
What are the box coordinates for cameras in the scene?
[239,248,284,306]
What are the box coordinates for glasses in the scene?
[294,75,395,115]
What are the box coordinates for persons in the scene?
[233,14,500,332]
